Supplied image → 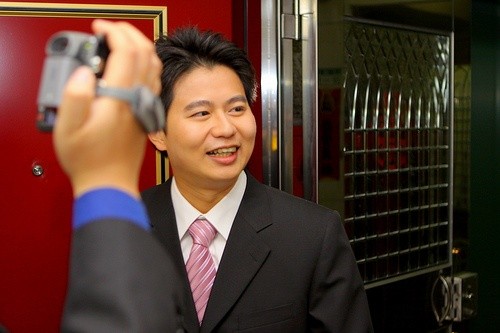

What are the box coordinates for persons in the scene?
[52,19,184,333]
[139,24,373,333]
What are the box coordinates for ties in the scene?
[185,219,217,327]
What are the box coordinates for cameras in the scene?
[37,29,110,133]
